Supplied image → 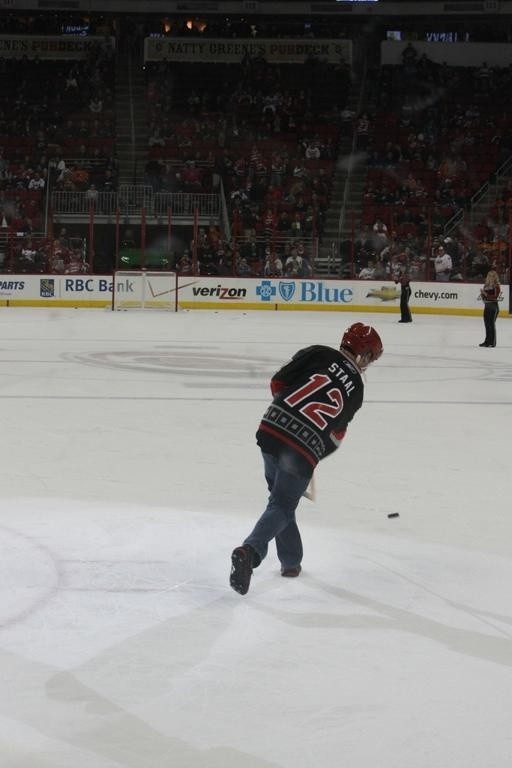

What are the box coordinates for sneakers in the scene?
[478,341,497,349]
[398,316,413,324]
[280,565,302,578]
[229,543,258,596]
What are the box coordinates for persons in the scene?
[392,262,412,323]
[474,269,501,346]
[229,321,384,596]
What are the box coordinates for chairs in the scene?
[1,41,512,283]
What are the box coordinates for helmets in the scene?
[339,322,385,374]
